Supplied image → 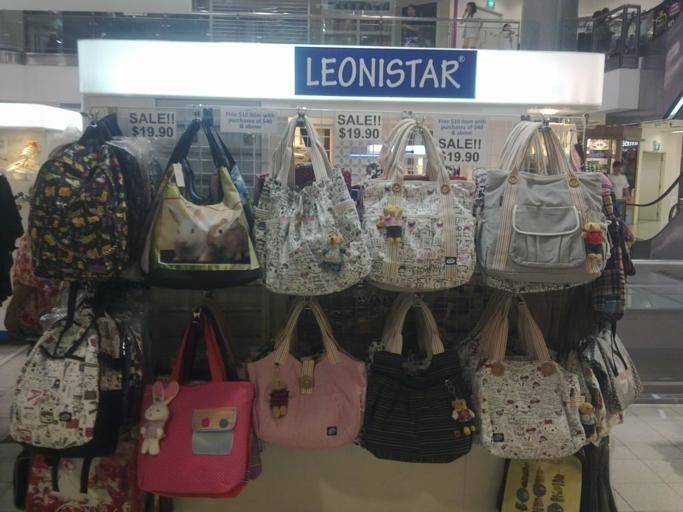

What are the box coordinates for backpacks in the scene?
[26,132,163,290]
[6,288,126,449]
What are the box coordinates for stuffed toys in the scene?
[140,380,178,454]
[379,204,405,243]
[452,396,477,435]
[581,221,605,266]
[317,232,352,274]
[579,402,600,444]
[267,377,290,418]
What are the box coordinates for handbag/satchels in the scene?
[356,117,479,296]
[11,443,148,512]
[134,308,256,500]
[455,293,642,458]
[134,117,264,291]
[354,297,480,467]
[233,297,370,452]
[493,452,599,512]
[471,121,641,319]
[247,108,376,297]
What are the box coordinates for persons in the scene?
[460,2,483,48]
[500,23,520,49]
[402,4,420,47]
[42,8,63,53]
[590,7,640,58]
[608,161,631,221]
